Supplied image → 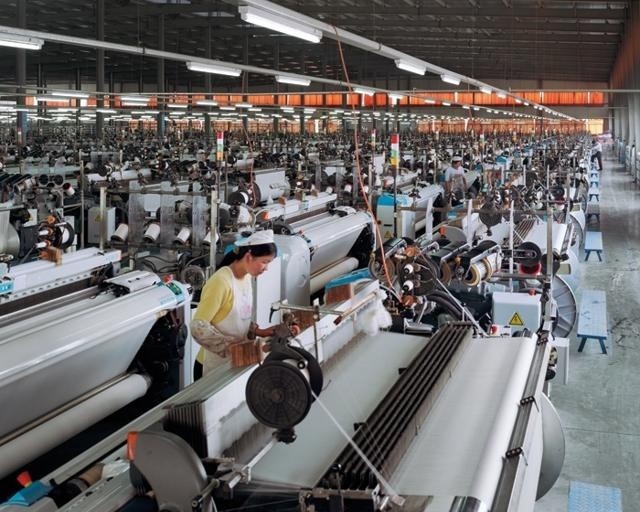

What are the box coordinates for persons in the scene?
[590,140,603,171]
[190,229,277,382]
[445,156,466,194]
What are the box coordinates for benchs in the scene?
[575,163,608,353]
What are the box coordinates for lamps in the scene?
[1,90,578,122]
[442,74,461,85]
[239,7,323,43]
[396,58,426,76]
[185,62,241,77]
[481,85,493,94]
[2,34,44,51]
[276,76,312,88]
[352,88,378,96]
